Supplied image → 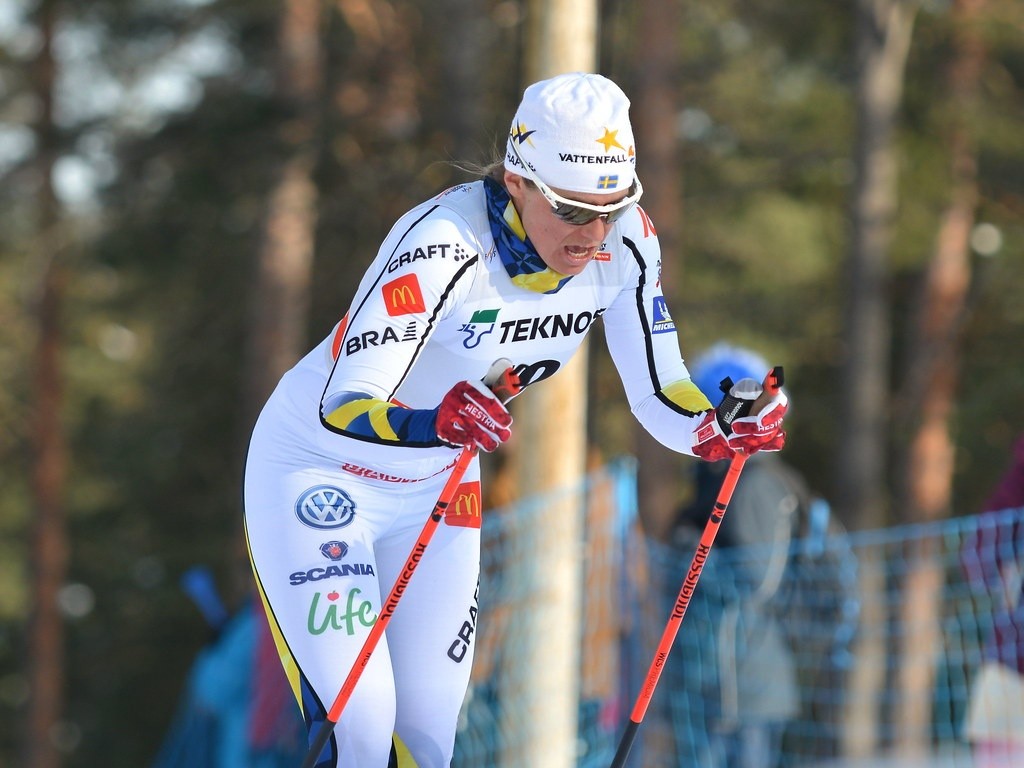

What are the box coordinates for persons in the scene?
[657,351,860,768]
[241,73,788,768]
[168,457,644,768]
[930,442,1024,768]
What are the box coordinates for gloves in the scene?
[434,379,515,455]
[692,375,789,463]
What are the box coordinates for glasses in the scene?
[508,133,644,225]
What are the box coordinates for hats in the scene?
[503,72,636,194]
[690,350,768,409]
